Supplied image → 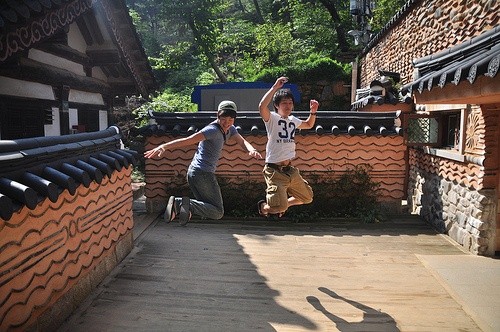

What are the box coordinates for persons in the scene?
[144,100,261,226]
[259,76,319,219]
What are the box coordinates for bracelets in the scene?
[311,112,316,115]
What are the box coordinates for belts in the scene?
[267,161,292,173]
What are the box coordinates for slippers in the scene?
[179,196,193,225]
[257,200,270,218]
[278,211,286,218]
[164,196,176,224]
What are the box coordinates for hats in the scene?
[217,100,237,113]
